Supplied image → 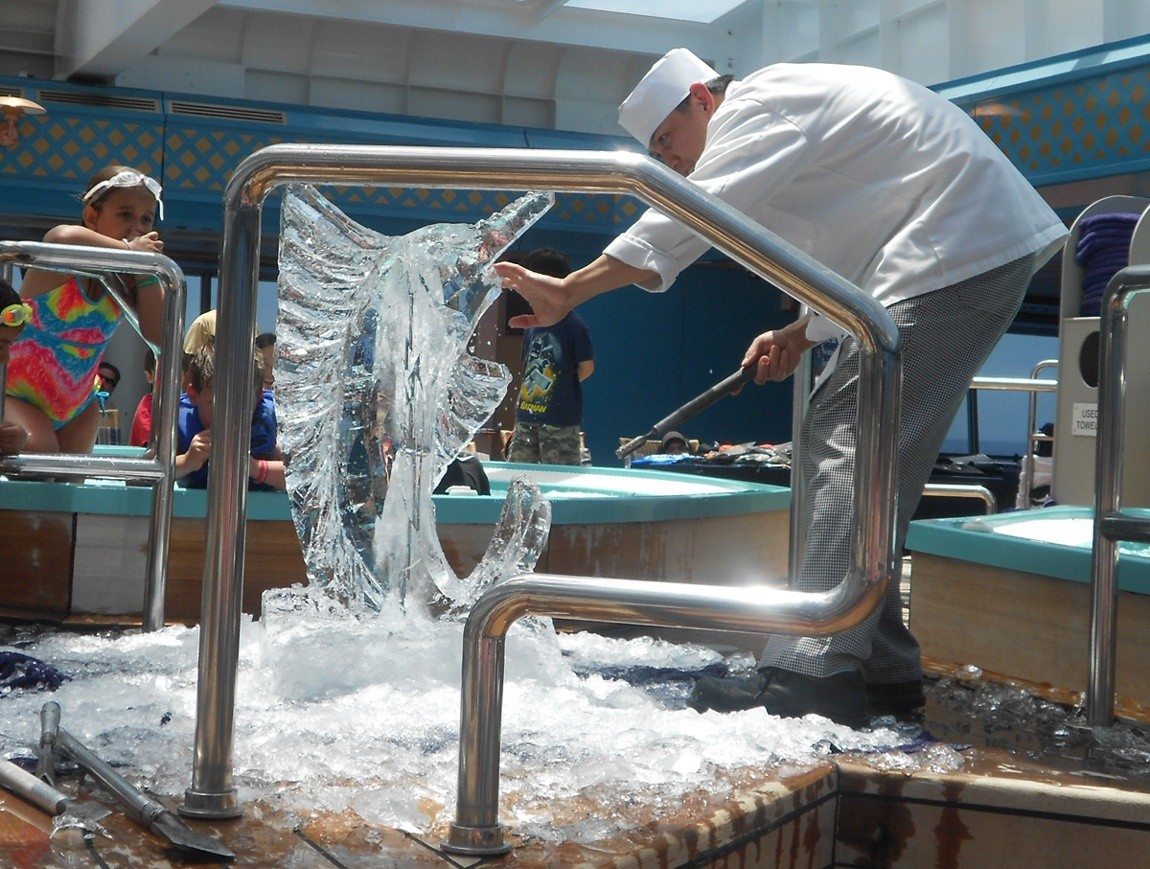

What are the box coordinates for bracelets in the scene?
[121,237,133,251]
[135,274,161,290]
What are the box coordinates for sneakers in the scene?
[864,680,927,712]
[686,667,873,730]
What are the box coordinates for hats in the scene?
[83,166,143,199]
[617,47,723,150]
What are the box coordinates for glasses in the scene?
[98,372,116,388]
[0,302,33,327]
[82,171,165,221]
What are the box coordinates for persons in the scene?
[3,162,164,485]
[431,452,492,497]
[94,361,120,399]
[0,278,35,455]
[492,47,1072,729]
[662,432,688,455]
[1038,422,1055,458]
[506,247,596,465]
[127,308,289,493]
